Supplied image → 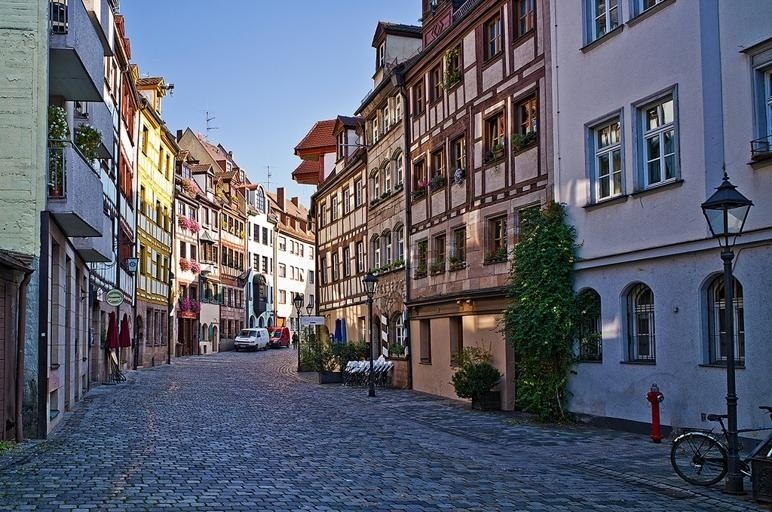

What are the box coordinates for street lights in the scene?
[701,172,755,474]
[306,301,313,317]
[293,293,303,368]
[362,269,379,391]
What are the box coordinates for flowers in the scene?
[76,123,104,165]
[47,105,68,188]
[179,179,245,312]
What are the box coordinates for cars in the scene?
[268,327,290,348]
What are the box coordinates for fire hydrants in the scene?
[646,383,664,442]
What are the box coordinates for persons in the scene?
[292,331,298,350]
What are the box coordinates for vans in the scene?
[233,327,270,352]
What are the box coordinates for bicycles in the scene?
[671,406,772,486]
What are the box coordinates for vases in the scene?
[50,185,62,196]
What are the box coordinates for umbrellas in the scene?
[119,312,131,370]
[106,310,120,378]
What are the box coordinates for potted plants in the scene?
[412,131,542,281]
[450,362,504,411]
[300,339,411,389]
